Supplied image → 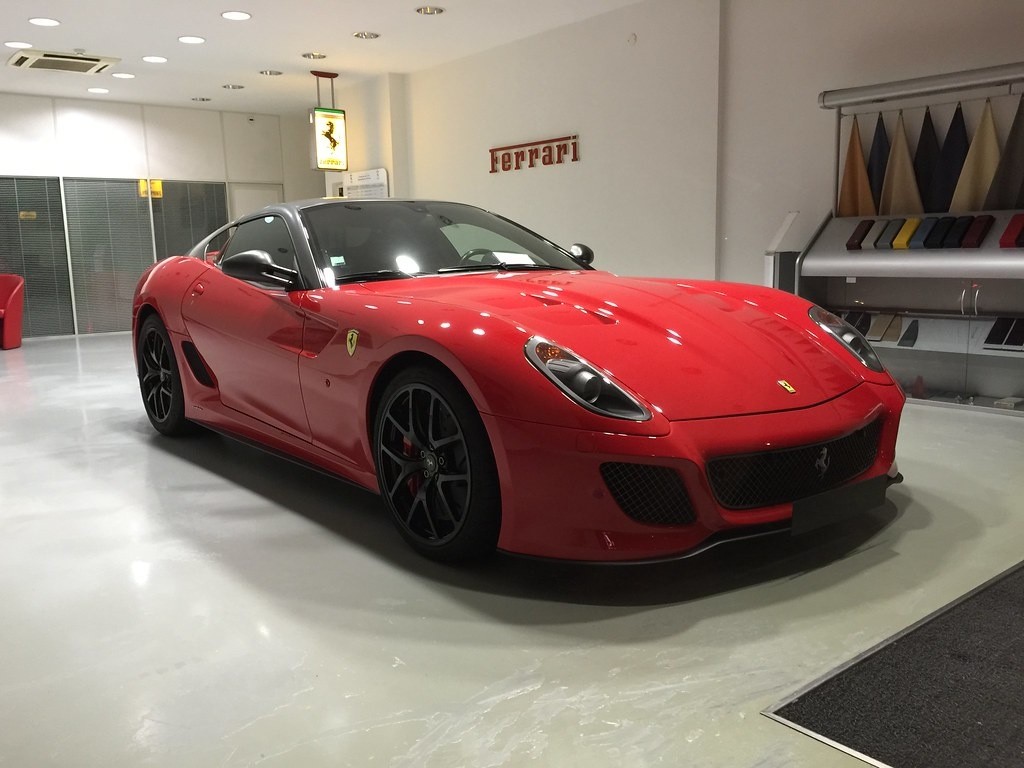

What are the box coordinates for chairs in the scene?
[0,273,25,349]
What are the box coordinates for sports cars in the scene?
[131,194,908,570]
